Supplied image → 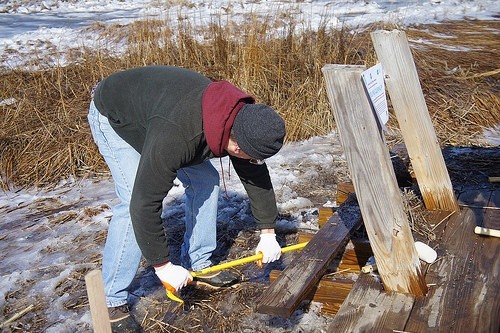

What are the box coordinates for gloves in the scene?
[255,234,281,267]
[153,262,193,294]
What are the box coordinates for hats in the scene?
[233,103,286,159]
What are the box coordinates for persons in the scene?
[86,64,287,333]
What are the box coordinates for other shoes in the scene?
[107,305,130,322]
[191,266,238,286]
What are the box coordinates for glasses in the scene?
[250,158,266,165]
